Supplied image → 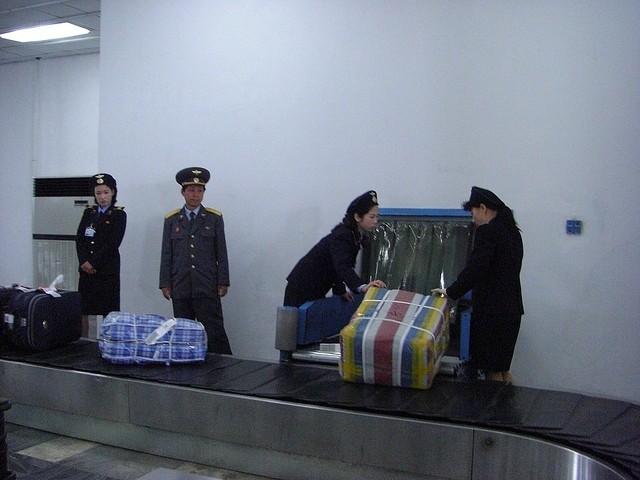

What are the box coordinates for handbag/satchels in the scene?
[98,311,208,366]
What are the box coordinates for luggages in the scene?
[1,283,82,351]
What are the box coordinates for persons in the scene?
[74,172,128,316]
[278,189,387,364]
[430,184,524,383]
[159,166,234,356]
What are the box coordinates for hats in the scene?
[90,174,116,191]
[176,167,210,187]
[470,186,505,210]
[346,190,379,216]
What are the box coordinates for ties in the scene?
[190,211,196,224]
[99,211,104,217]
